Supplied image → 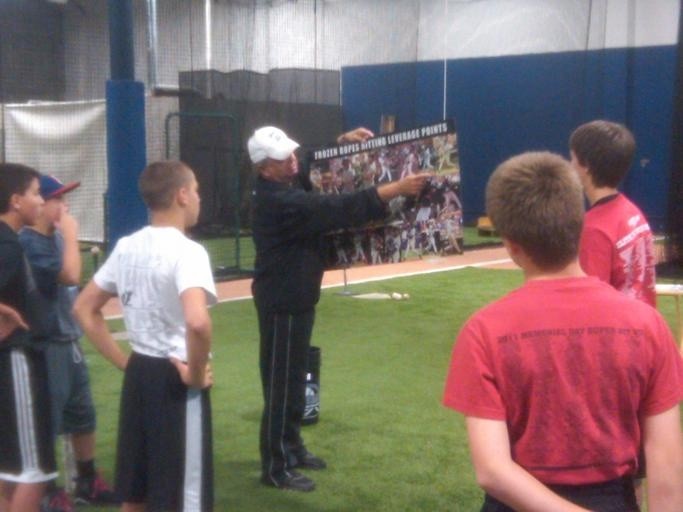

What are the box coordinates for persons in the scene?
[0,161,61,512]
[310,122,464,269]
[568,120,659,309]
[439,149,682,512]
[70,161,218,512]
[21,172,118,512]
[246,126,431,493]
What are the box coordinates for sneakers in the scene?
[39,487,75,512]
[280,473,315,492]
[297,451,326,469]
[72,475,121,506]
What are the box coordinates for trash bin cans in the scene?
[302,345,321,427]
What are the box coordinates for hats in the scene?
[39,174,79,200]
[247,126,301,164]
